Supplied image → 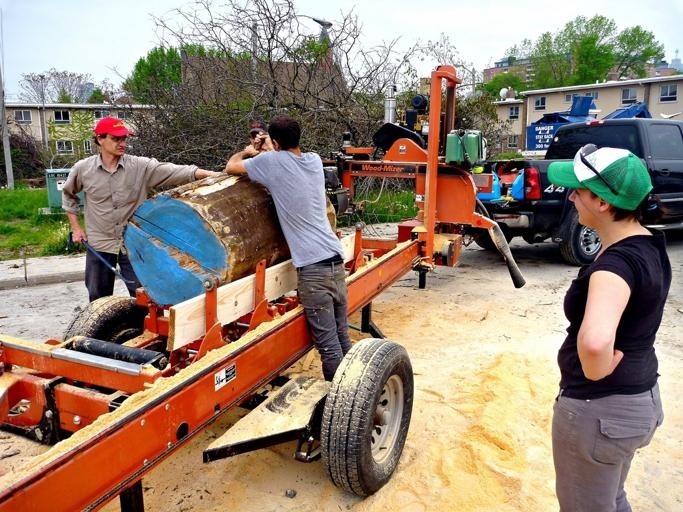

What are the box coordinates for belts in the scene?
[296,259,344,272]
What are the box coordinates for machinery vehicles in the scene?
[522,96,652,160]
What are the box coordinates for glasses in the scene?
[573,143,619,198]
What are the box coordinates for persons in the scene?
[246,126,268,149]
[58,114,226,301]
[222,115,356,383]
[543,144,673,510]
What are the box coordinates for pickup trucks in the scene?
[468,115,683,267]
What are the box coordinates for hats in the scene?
[248,120,267,135]
[94,117,135,139]
[547,147,654,212]
